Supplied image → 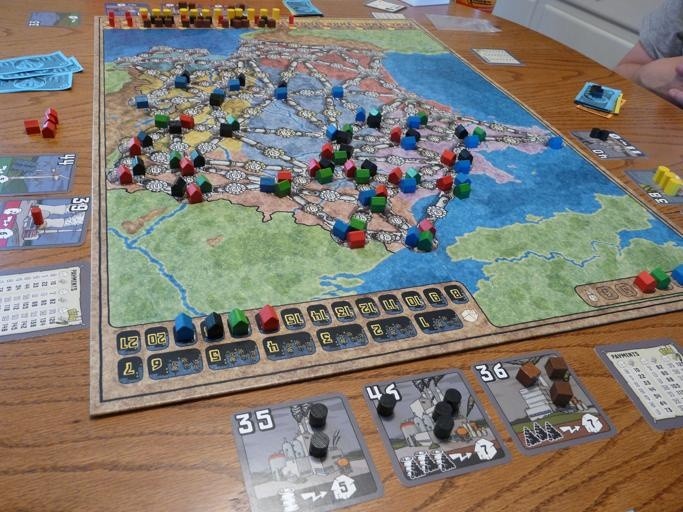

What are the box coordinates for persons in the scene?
[611,1,683,111]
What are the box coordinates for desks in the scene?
[0,0,681,512]
